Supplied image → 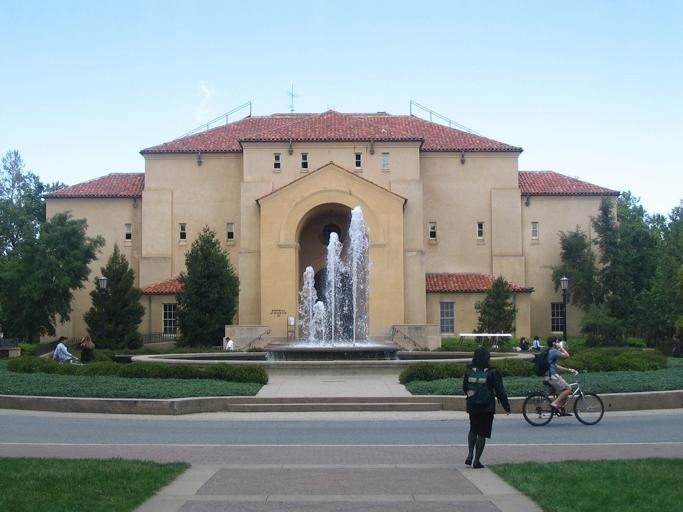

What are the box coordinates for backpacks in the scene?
[466,366,496,416]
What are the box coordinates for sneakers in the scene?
[465,457,485,468]
[531,348,556,376]
[549,403,572,416]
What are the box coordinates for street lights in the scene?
[559,273,569,340]
[98,276,108,348]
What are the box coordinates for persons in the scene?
[52,336,83,365]
[461,347,510,469]
[532,335,543,351]
[224,337,232,351]
[519,337,529,350]
[557,337,566,351]
[541,335,580,417]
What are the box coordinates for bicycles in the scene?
[522,369,604,426]
